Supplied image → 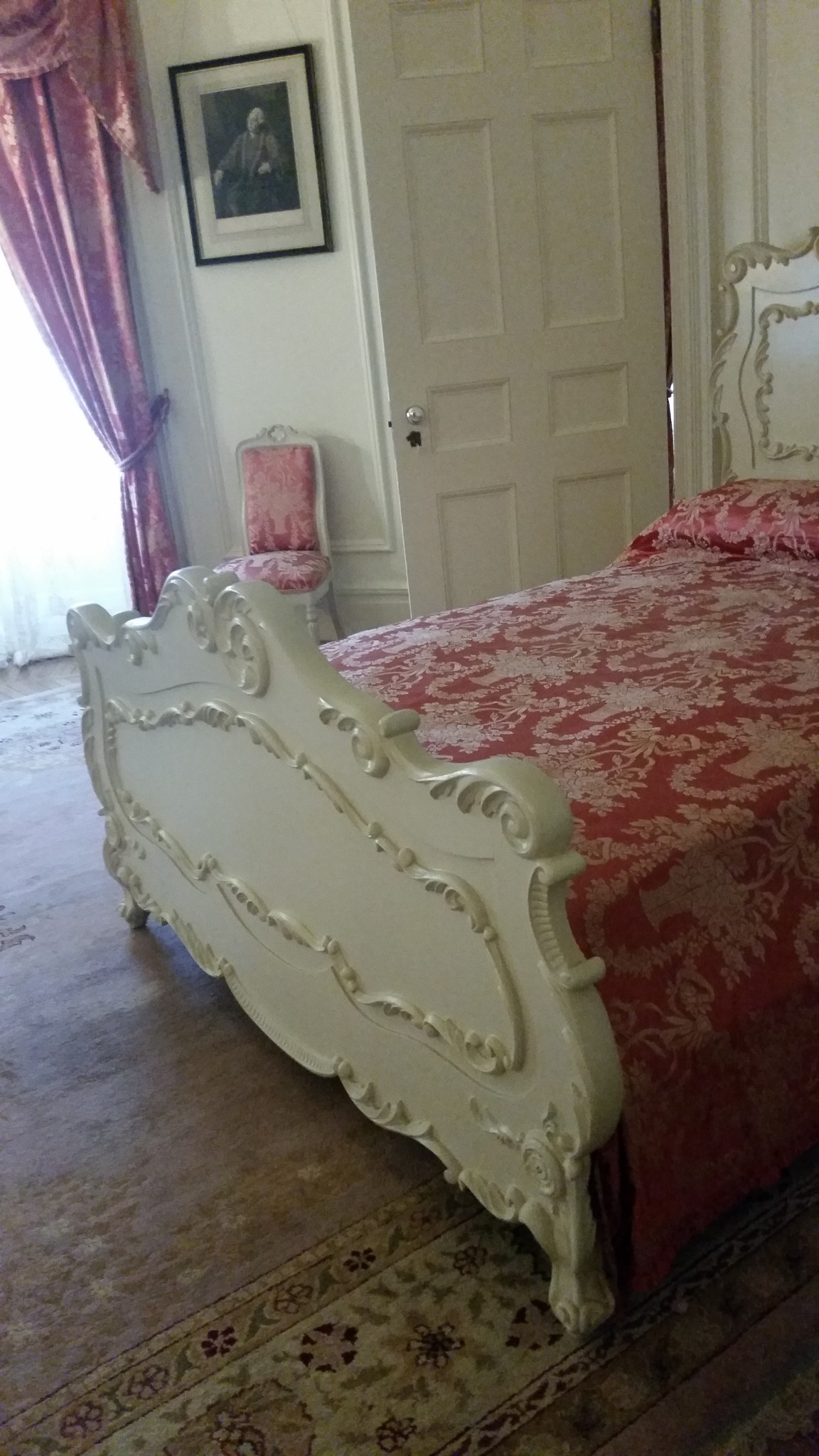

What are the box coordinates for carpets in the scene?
[0,1152,819,1456]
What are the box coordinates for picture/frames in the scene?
[168,44,334,267]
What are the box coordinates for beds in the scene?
[68,228,819,1339]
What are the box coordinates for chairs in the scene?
[212,425,346,646]
